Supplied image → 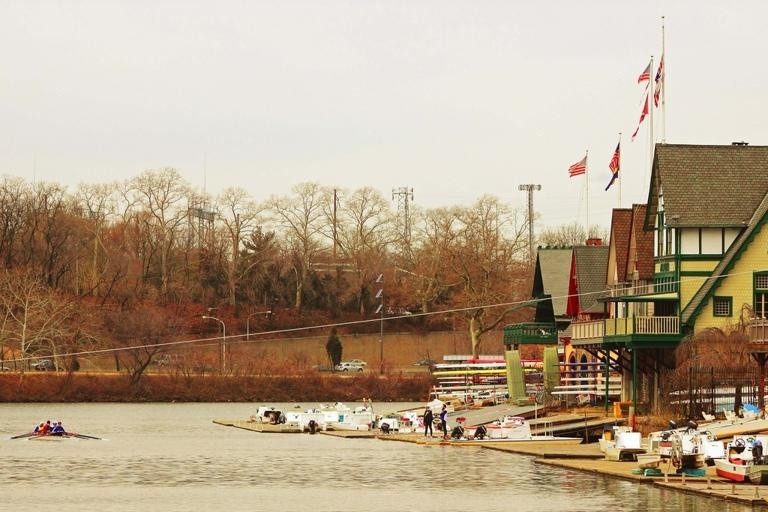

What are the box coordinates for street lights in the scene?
[518,183,541,264]
[202,315,226,376]
[749,350,767,411]
[246,310,271,340]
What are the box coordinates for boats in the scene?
[254,396,532,439]
[598,424,768,485]
[30,435,71,441]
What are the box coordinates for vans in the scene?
[384,306,412,315]
[153,353,187,369]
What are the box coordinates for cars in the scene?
[350,359,368,366]
[412,358,437,366]
[0,366,11,372]
[311,365,334,371]
[193,363,215,374]
[33,360,64,372]
[335,363,363,372]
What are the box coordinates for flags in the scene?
[569,156,586,177]
[631,63,651,140]
[654,56,662,111]
[605,141,620,192]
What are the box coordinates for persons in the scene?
[423,405,433,437]
[33,419,66,436]
[439,403,448,438]
